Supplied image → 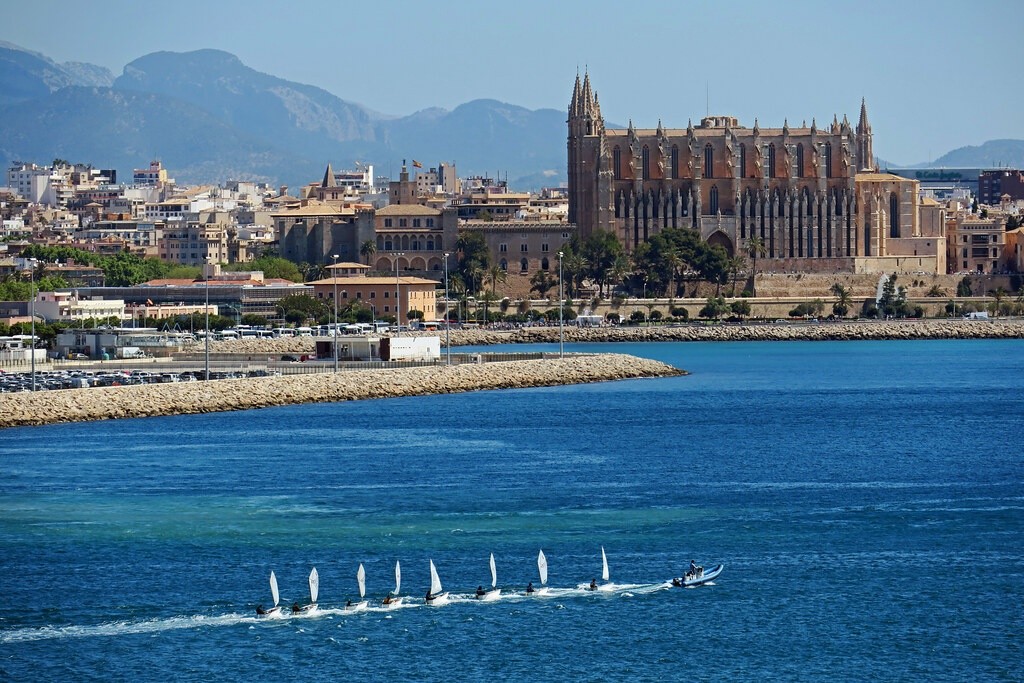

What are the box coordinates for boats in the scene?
[661,563,724,589]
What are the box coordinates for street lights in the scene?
[128,252,652,337]
[556,251,565,359]
[204,256,211,379]
[442,253,450,365]
[330,254,341,372]
[27,257,38,392]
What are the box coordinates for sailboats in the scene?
[255,542,652,621]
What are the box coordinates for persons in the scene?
[384,594,391,604]
[425,590,434,600]
[347,599,353,606]
[527,582,535,592]
[292,601,304,612]
[256,604,267,615]
[690,559,701,574]
[476,586,485,595]
[590,578,596,589]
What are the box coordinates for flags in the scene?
[412,159,422,169]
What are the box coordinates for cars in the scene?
[908,267,1024,280]
[0,295,1024,393]
